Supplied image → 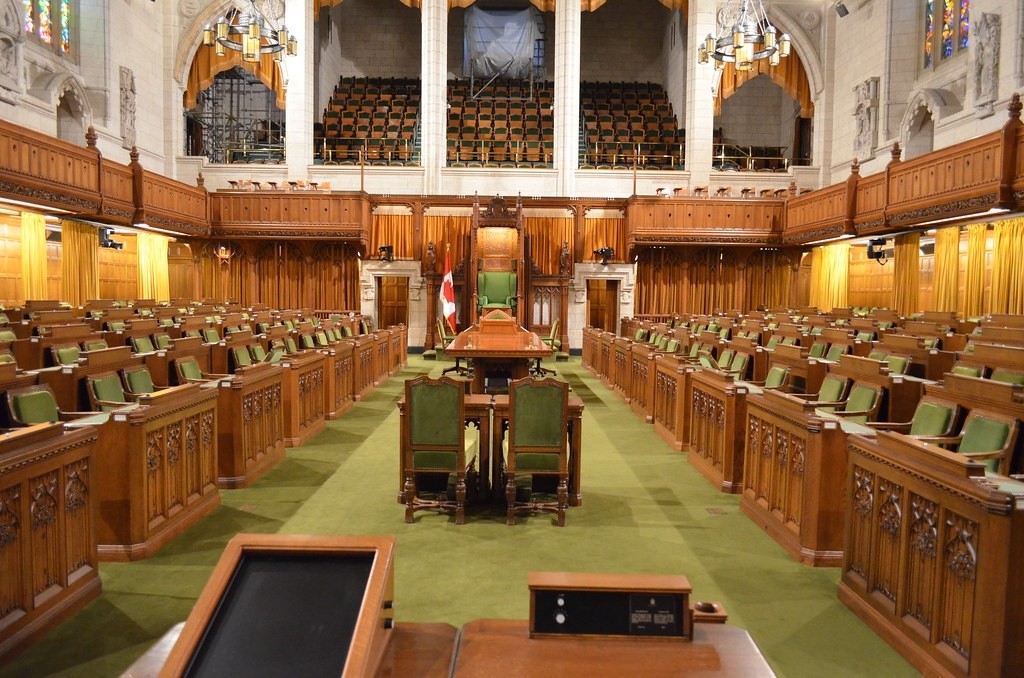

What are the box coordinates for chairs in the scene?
[436,319,472,376]
[0,302,371,430]
[231,74,789,173]
[499,375,571,527]
[633,306,1023,478]
[403,375,476,525]
[475,271,518,313]
[528,319,560,378]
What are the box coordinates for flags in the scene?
[439,251,457,335]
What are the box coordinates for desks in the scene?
[580,305,1024,453]
[684,368,748,495]
[739,387,876,567]
[115,618,776,677]
[835,428,1024,677]
[490,394,584,508]
[0,421,103,666]
[397,394,493,506]
[444,321,554,394]
[0,298,408,420]
[65,380,222,563]
[198,361,287,490]
[279,348,326,447]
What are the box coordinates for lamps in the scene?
[202,0,297,63]
[698,0,791,73]
